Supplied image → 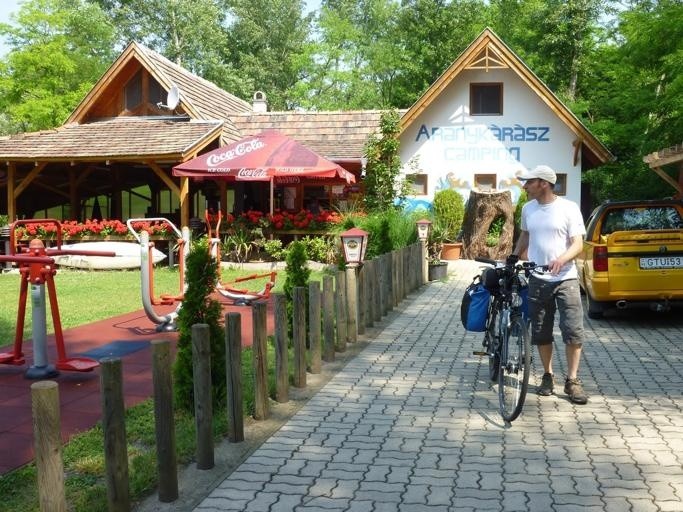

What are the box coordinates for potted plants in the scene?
[436,189,463,260]
[425,226,448,280]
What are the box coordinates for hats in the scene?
[517,166,557,185]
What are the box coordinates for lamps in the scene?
[339,227,369,263]
[414,219,432,240]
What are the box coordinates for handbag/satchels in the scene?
[461,275,491,332]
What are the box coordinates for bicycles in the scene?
[472,255,553,422]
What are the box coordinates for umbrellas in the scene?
[172,128,356,240]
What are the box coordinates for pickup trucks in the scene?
[573,199,682,320]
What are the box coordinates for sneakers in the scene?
[537,374,555,396]
[563,378,587,403]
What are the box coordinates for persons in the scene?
[506,165,588,404]
